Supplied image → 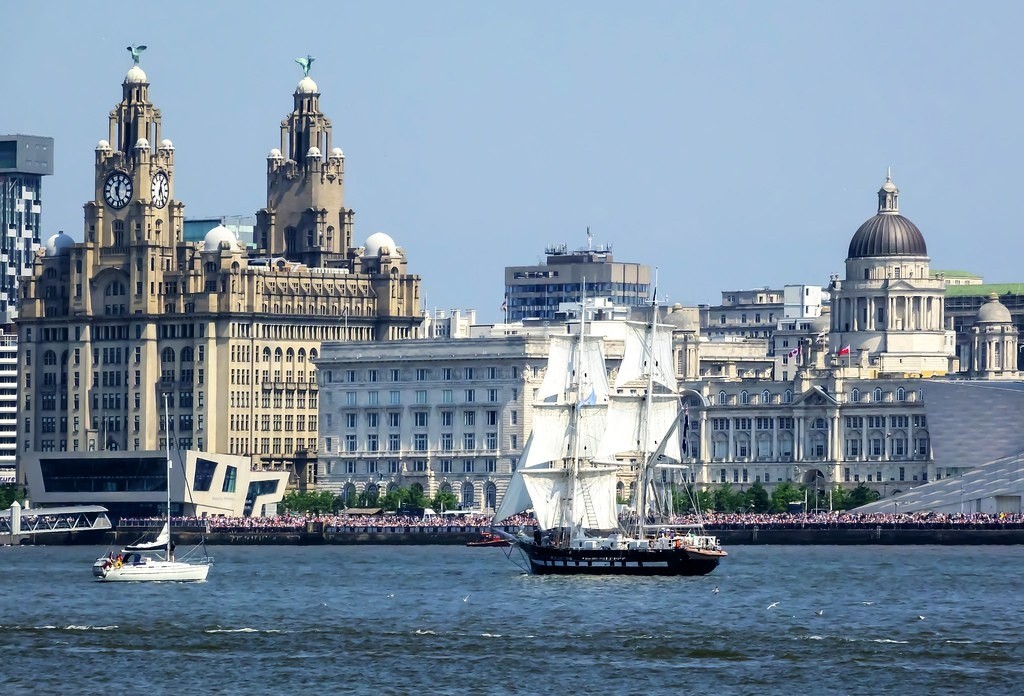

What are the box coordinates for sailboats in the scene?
[92,393,213,582]
[490,265,728,575]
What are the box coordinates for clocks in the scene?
[151,171,169,209]
[103,171,134,210]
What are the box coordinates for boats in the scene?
[467,532,511,547]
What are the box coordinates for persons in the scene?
[0,515,75,522]
[109,509,1024,568]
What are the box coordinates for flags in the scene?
[789,346,801,358]
[838,345,849,355]
